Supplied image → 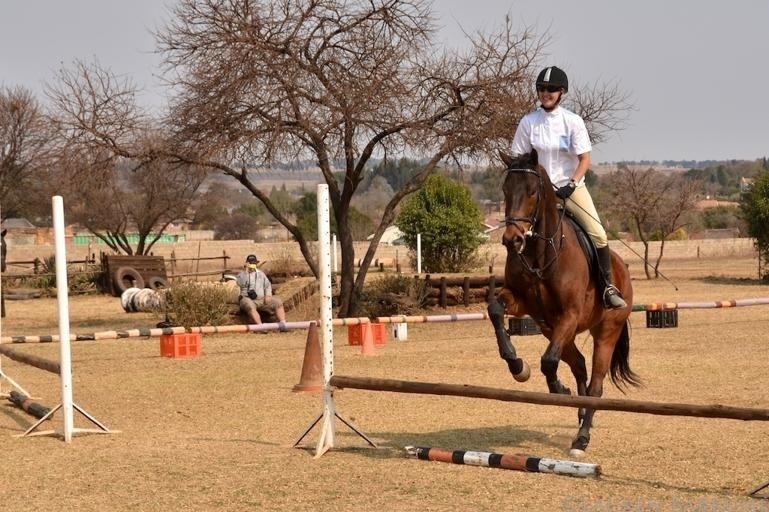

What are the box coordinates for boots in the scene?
[594,243,629,310]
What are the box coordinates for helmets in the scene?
[536,65,569,90]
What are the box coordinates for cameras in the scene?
[247,289,257,300]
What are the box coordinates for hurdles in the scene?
[293,182,769,459]
[2,195,110,443]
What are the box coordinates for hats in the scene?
[246,254,260,264]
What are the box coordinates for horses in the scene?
[0,229,7,317]
[487,148,649,459]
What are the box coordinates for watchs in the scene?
[570,179,579,187]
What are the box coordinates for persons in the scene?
[510,65,628,310]
[236,255,290,334]
[1,227,7,317]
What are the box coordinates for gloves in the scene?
[555,177,580,199]
